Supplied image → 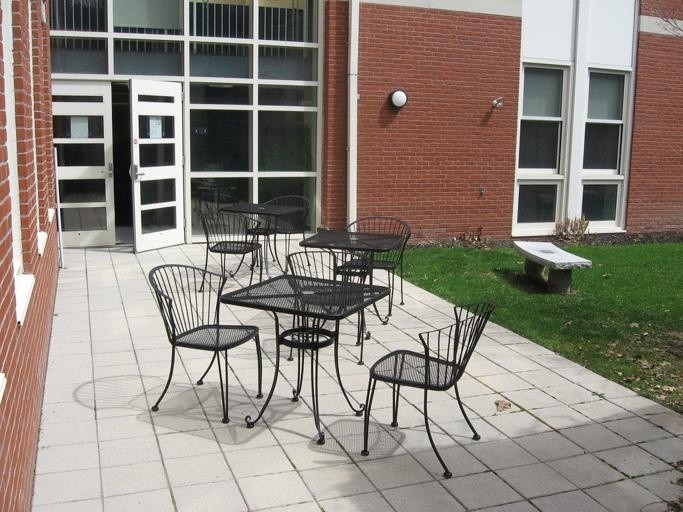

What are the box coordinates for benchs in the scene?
[512,238,593,296]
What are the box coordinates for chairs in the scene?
[361,302,496,479]
[197,197,410,344]
[147,265,264,424]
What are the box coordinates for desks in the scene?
[218,275,390,446]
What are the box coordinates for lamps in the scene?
[389,88,409,110]
